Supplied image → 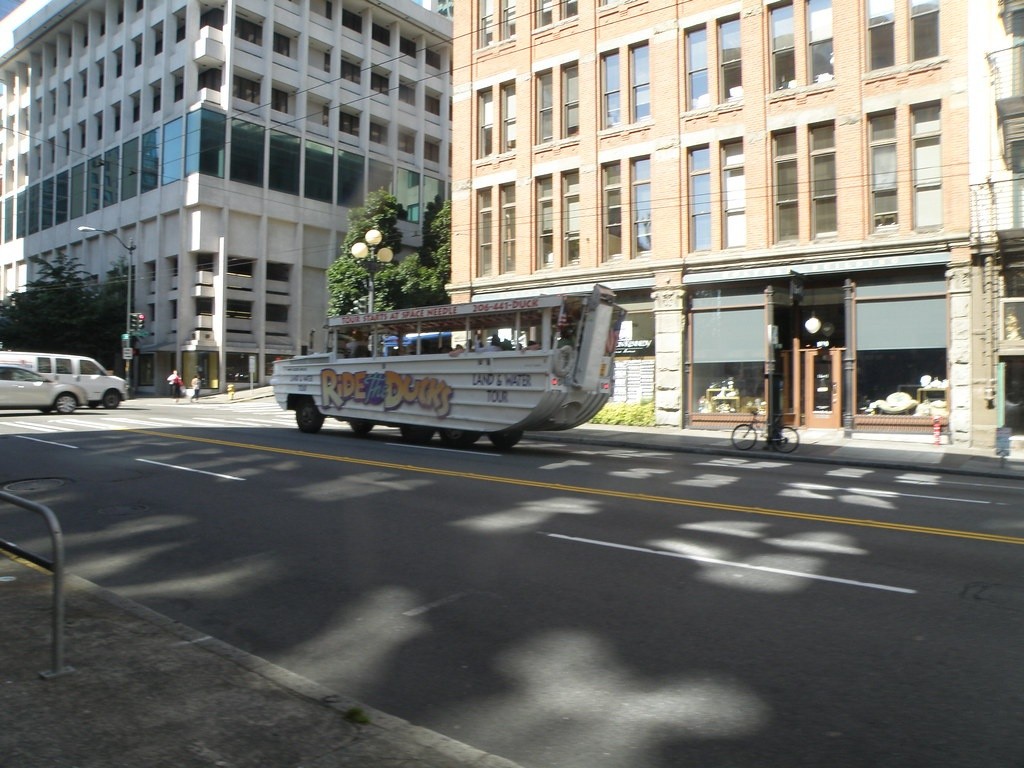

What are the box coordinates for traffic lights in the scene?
[130,313,145,336]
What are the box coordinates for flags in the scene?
[558,302,569,325]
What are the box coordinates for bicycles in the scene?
[731,411,799,453]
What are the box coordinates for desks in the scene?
[917,388,947,404]
[705,388,739,408]
[710,395,741,413]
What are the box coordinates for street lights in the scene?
[351,229,393,313]
[78,226,136,390]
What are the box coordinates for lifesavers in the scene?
[553,345,575,377]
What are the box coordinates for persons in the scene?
[345,330,512,358]
[167,370,201,400]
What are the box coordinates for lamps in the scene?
[804,288,822,334]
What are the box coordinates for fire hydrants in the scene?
[227,384,234,399]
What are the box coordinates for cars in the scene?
[0,365,87,415]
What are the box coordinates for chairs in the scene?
[875,392,919,415]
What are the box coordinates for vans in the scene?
[0,351,126,409]
